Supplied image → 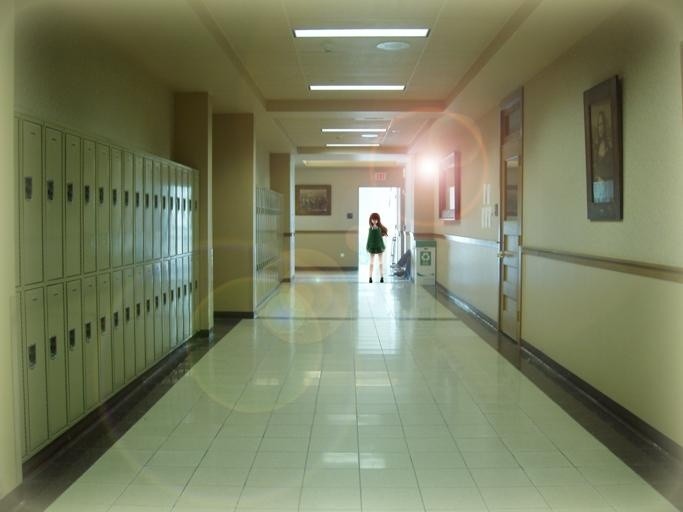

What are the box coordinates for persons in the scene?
[590,113,614,203]
[365,213,387,283]
[388,250,409,279]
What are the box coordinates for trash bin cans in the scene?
[414,239,436,287]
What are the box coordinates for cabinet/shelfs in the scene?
[255,186,284,314]
[16,108,199,465]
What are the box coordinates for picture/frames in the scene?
[437,151,459,221]
[295,185,331,216]
[582,73,623,221]
